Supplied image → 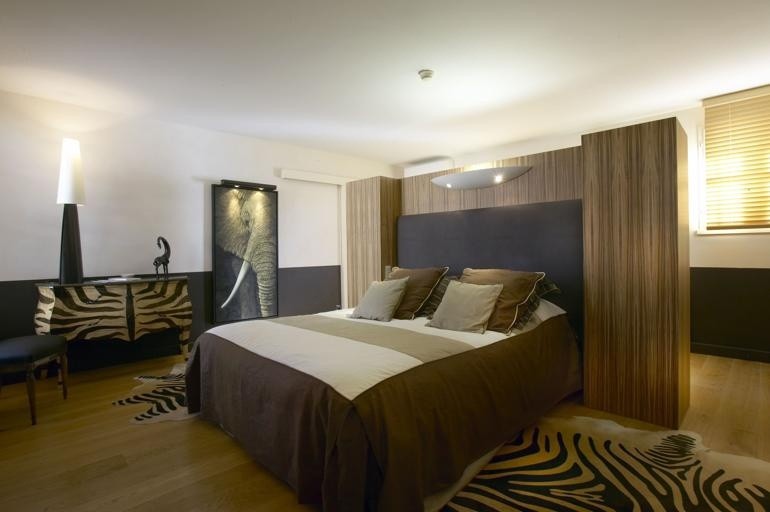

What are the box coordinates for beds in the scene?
[184,201,583,512]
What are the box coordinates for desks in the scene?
[34,274,191,379]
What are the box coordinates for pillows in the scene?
[350,264,555,333]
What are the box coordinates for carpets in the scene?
[112,362,194,423]
[427,414,770,512]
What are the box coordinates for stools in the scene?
[0,336,69,425]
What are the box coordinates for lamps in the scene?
[429,165,533,191]
[57,139,86,284]
